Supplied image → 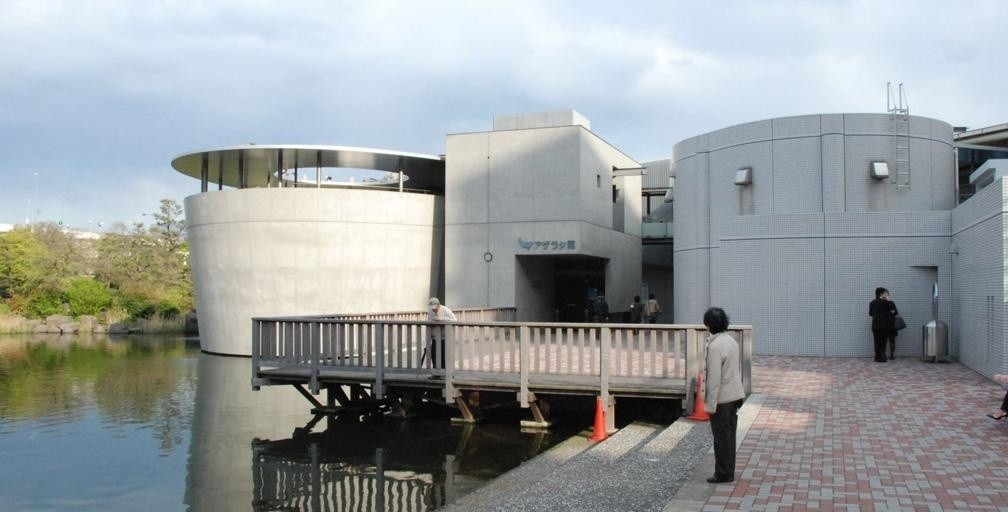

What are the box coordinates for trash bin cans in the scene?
[923,319,948,364]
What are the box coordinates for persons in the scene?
[643,294,662,324]
[869,287,891,362]
[986,374,1008,420]
[426,297,458,368]
[701,307,745,483]
[883,288,898,362]
[630,295,643,335]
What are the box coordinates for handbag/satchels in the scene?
[892,314,906,331]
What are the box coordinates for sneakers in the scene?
[875,356,896,363]
[707,476,734,483]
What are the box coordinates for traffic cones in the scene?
[587,393,609,441]
[685,371,712,422]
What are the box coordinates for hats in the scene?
[428,296,440,310]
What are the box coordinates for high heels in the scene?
[985,410,1008,422]
